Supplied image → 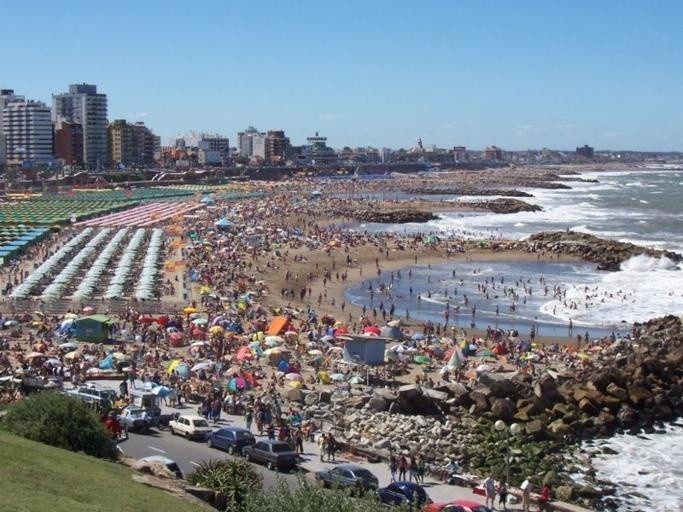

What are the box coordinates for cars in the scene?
[241,439,298,470]
[204,426,256,455]
[169,415,213,441]
[116,406,153,432]
[314,464,493,511]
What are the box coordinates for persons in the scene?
[539,482,550,512]
[498,476,508,511]
[520,476,534,512]
[1,178,683,485]
[483,472,497,509]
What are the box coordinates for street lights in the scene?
[494,419,521,489]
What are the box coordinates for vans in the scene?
[67,386,117,411]
